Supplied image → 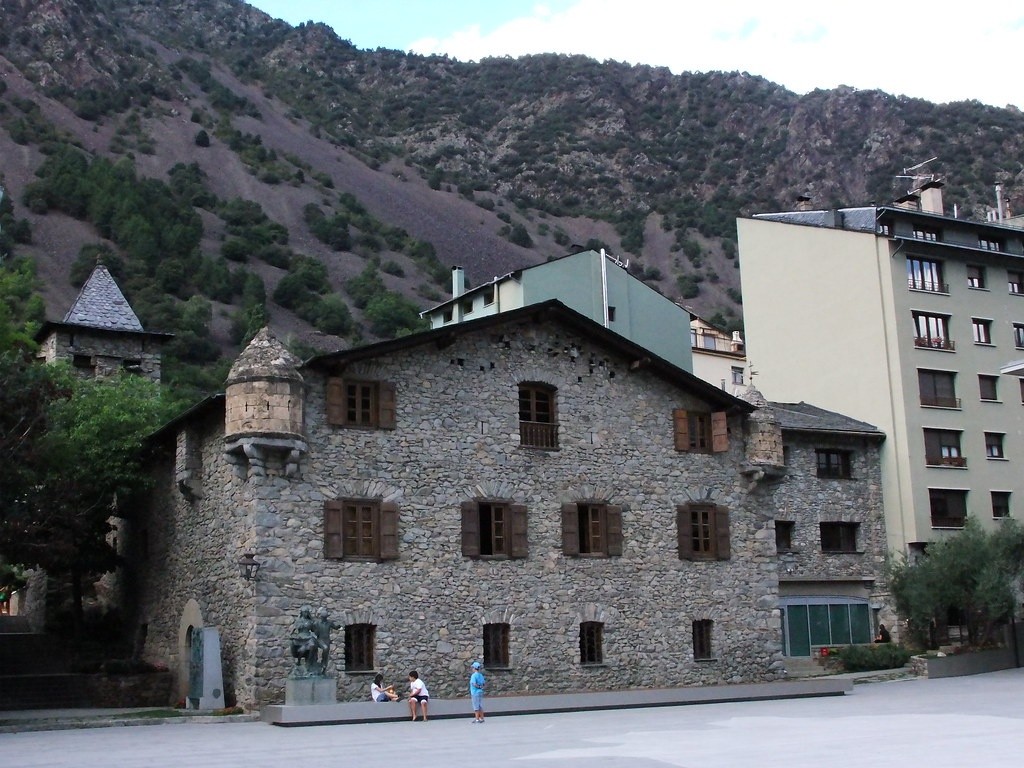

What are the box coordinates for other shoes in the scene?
[472,719,485,723]
[396,693,405,702]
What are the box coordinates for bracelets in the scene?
[479,685,480,689]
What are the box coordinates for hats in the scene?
[471,661,481,670]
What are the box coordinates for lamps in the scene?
[238,552,261,581]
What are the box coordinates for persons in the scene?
[313,610,343,670]
[407,671,429,722]
[371,674,398,702]
[290,606,317,666]
[874,624,891,643]
[470,662,485,723]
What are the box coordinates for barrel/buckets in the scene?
[820,648,828,657]
